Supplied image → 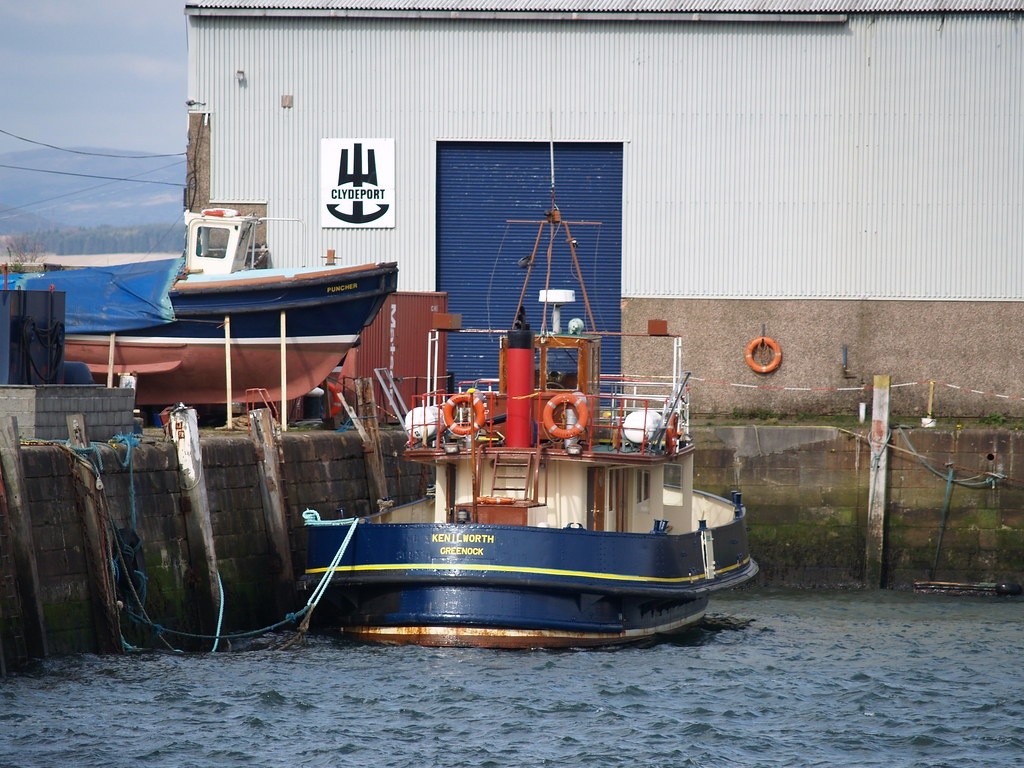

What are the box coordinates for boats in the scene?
[1,248,400,403]
[294,108,759,650]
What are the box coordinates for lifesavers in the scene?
[202,207,241,218]
[319,380,342,416]
[477,497,515,504]
[540,393,588,440]
[441,393,486,438]
[743,337,784,374]
[665,413,680,454]
